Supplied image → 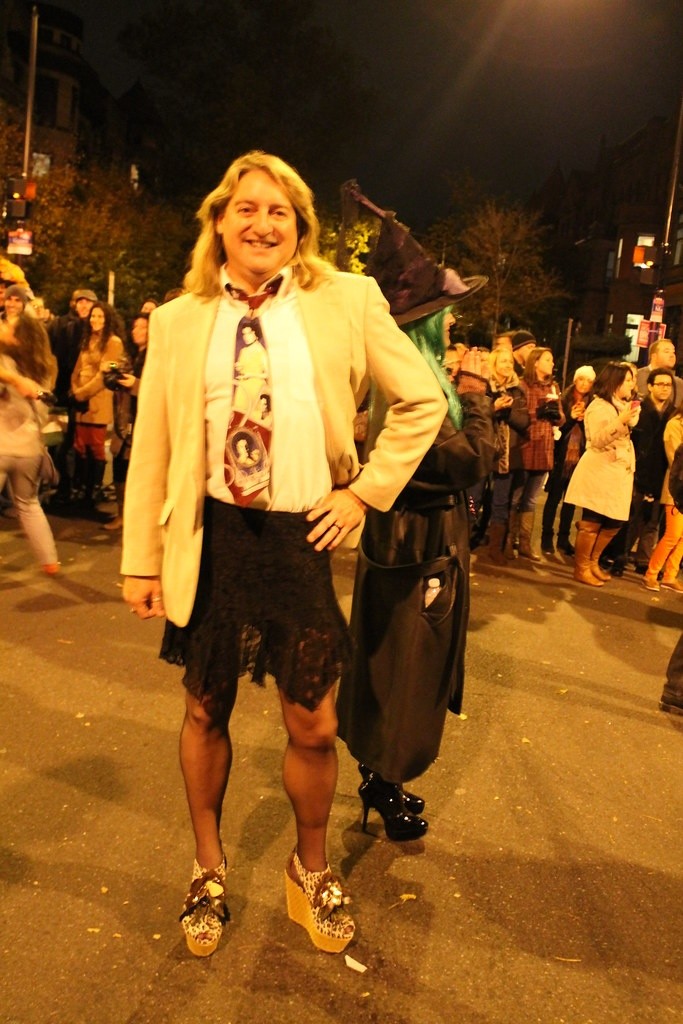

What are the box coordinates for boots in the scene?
[590,526,620,580]
[574,519,604,587]
[503,511,515,560]
[516,511,540,561]
[489,523,508,565]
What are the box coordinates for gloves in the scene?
[536,401,561,422]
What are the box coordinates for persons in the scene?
[119,150,449,957]
[0,256,188,579]
[658,443,683,716]
[440,330,683,594]
[334,218,499,842]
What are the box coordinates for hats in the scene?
[69,289,98,307]
[574,364,596,383]
[510,330,535,350]
[335,180,489,325]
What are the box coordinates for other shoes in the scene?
[541,530,554,555]
[638,576,659,591]
[46,563,61,574]
[556,532,576,555]
[610,560,624,576]
[657,686,683,718]
[103,515,124,531]
[661,583,683,593]
[634,565,664,581]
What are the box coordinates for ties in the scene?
[226,273,285,509]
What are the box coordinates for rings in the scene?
[334,524,341,530]
[152,598,161,602]
[130,608,136,613]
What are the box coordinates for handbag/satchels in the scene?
[43,450,60,487]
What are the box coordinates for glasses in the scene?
[649,380,673,390]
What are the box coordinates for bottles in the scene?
[426,578,445,608]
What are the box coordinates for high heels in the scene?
[283,847,355,954]
[357,760,426,815]
[357,772,427,841]
[179,856,229,957]
